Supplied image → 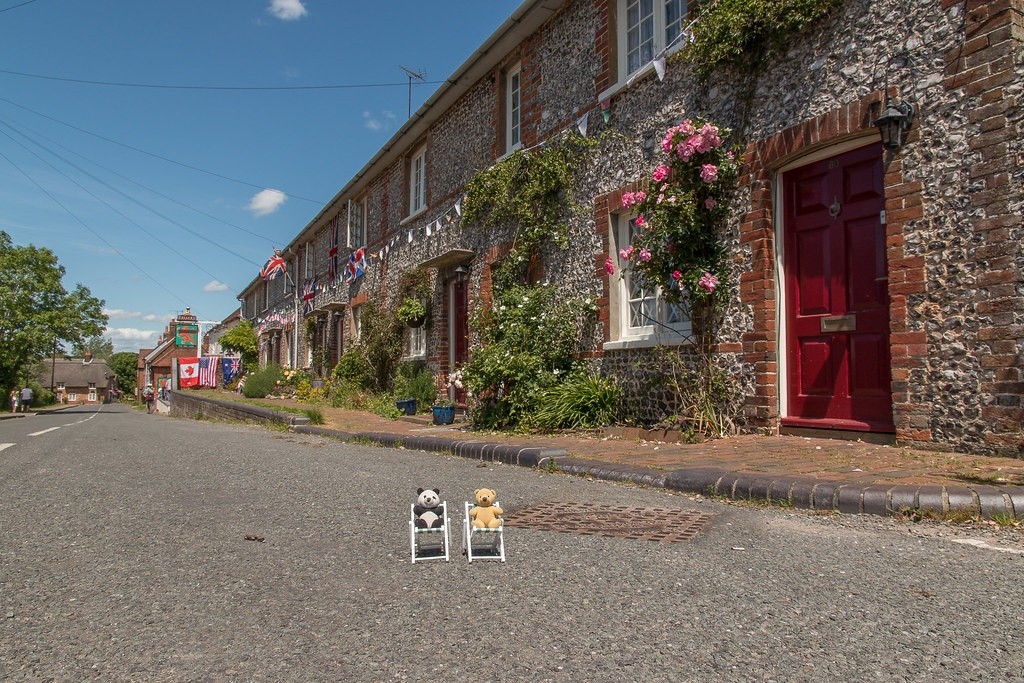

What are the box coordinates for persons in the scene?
[20,385,33,413]
[9,386,20,413]
[143,383,154,414]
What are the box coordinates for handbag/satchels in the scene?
[144,394,154,401]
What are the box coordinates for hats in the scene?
[147,384,153,386]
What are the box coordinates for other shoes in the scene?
[27,411,29,413]
[21,411,24,413]
[147,412,153,414]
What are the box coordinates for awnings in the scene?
[108,388,122,395]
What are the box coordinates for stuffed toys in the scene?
[469,488,503,528]
[413,487,444,529]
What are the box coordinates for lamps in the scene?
[873,94,915,150]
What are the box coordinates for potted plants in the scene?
[433,400,457,426]
[393,375,416,416]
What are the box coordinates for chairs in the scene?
[462,500,507,563]
[408,501,453,565]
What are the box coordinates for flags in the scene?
[346,249,367,284]
[259,254,286,281]
[222,357,241,385]
[199,357,219,387]
[302,278,315,315]
[178,357,199,387]
